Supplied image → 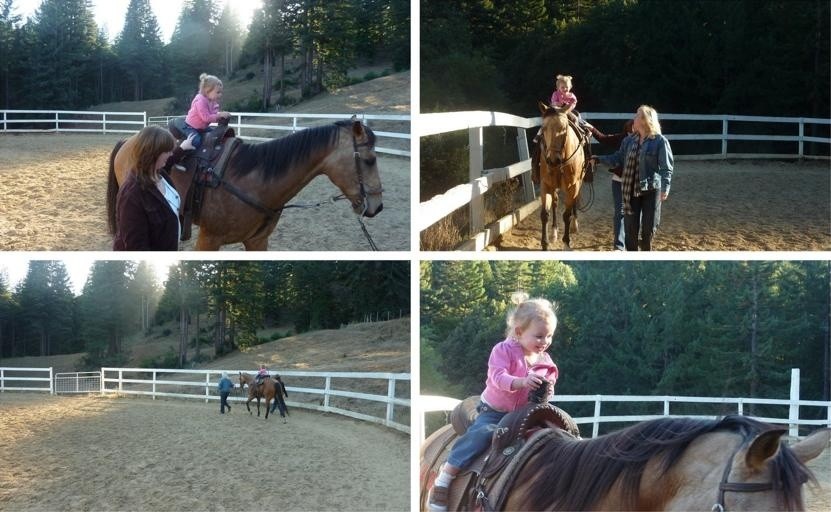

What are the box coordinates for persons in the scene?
[254,364,267,383]
[580,118,643,250]
[269,373,288,417]
[183,72,233,155]
[110,125,199,250]
[217,372,235,414]
[532,73,591,143]
[424,291,560,512]
[586,103,676,250]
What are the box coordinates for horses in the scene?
[538,100,590,250]
[105,114,384,251]
[239,372,290,423]
[420,414,831,512]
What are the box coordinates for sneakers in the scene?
[173,160,186,172]
[533,135,540,143]
[425,480,449,512]
